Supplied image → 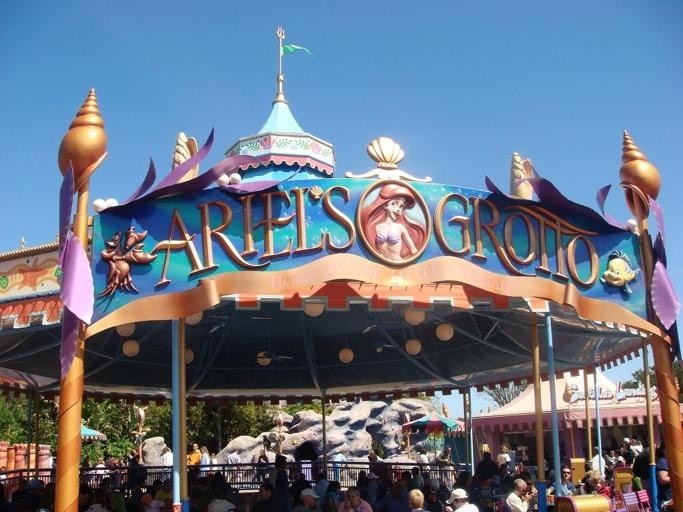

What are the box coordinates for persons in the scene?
[360,182,426,264]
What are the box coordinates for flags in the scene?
[281,35,314,57]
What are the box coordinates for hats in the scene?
[301,488,320,499]
[29,480,43,489]
[445,488,469,505]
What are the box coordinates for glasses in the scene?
[562,470,572,474]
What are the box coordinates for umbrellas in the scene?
[401,412,465,458]
[80,415,108,449]
